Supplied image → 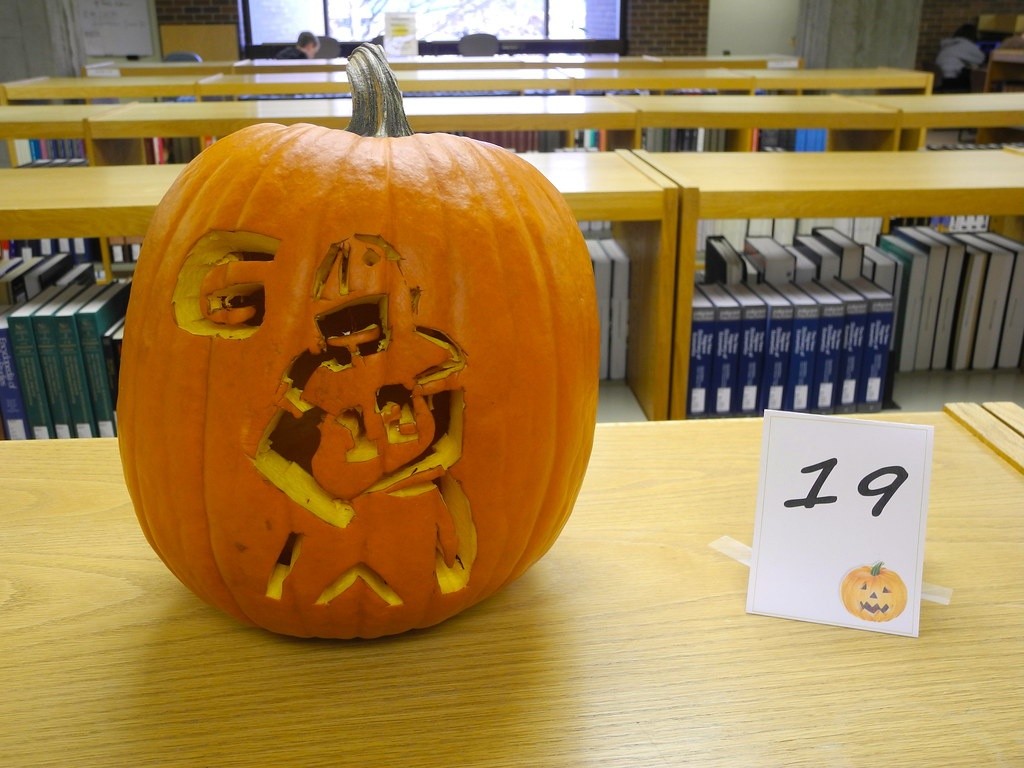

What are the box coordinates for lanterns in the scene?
[117,124,601,639]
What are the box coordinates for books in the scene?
[14,87,1024,167]
[0,237,141,442]
[577,219,634,382]
[687,215,1024,415]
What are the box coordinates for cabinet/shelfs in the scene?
[1,48,1024,766]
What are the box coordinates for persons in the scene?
[278,32,320,59]
[936,26,1024,93]
